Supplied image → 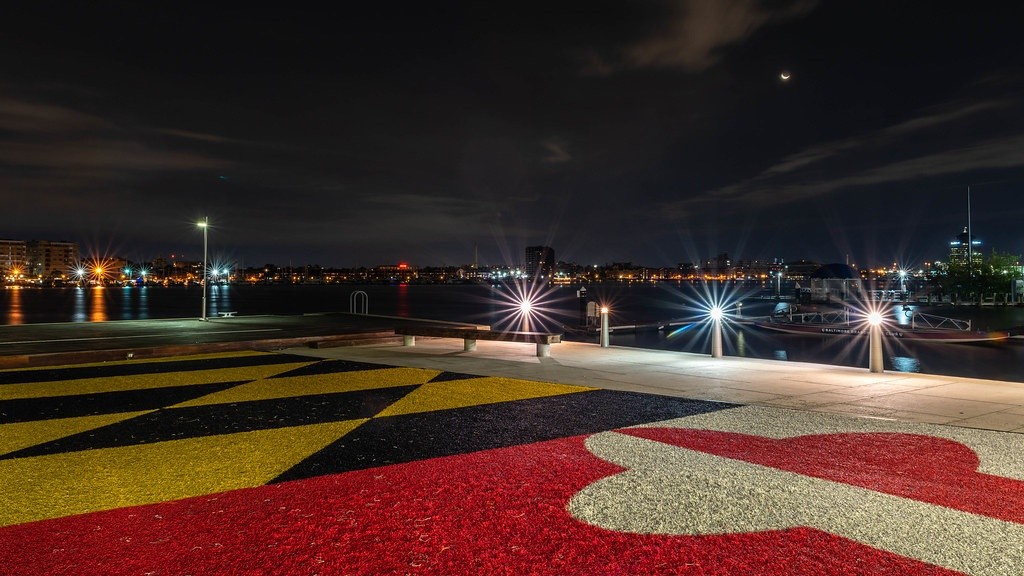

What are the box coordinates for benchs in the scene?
[217,312,237,318]
[395,323,563,357]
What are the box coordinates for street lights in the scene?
[768,259,789,295]
[897,268,908,300]
[195,216,213,326]
[515,298,532,343]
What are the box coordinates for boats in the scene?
[756,305,1010,342]
